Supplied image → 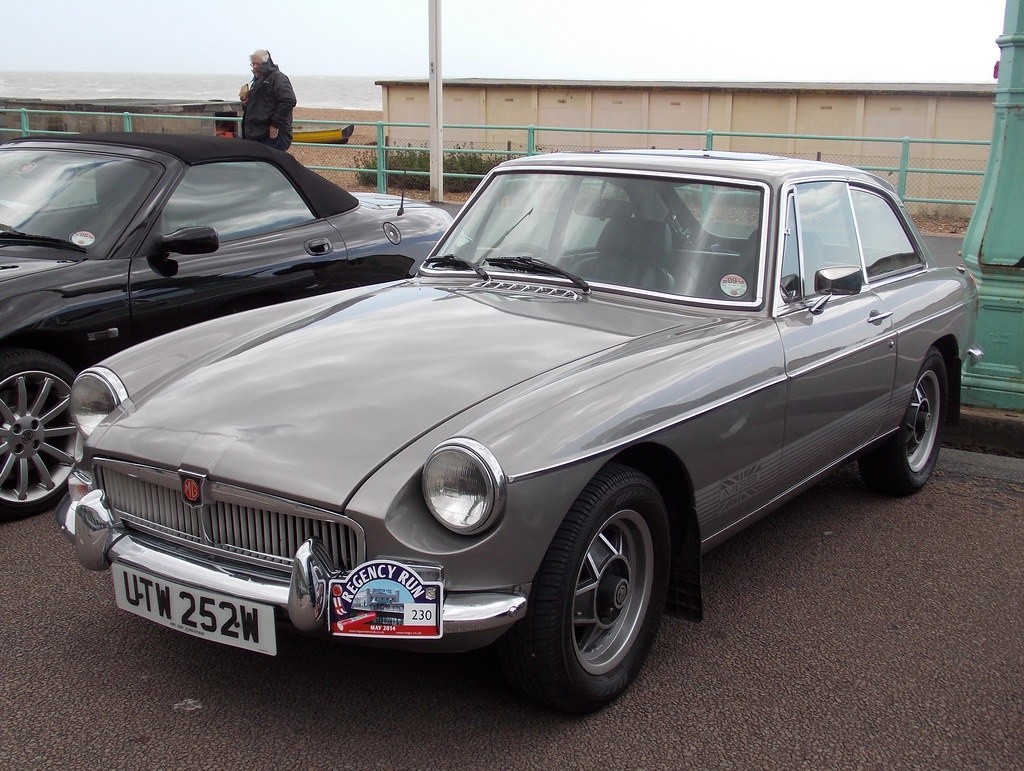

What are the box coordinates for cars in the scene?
[0,131,457,523]
[54,148,986,719]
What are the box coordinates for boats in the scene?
[291,122,355,145]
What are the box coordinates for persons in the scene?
[235,48,296,151]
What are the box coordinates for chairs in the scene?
[573,215,676,296]
[721,228,825,304]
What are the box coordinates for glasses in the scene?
[250,62,261,67]
